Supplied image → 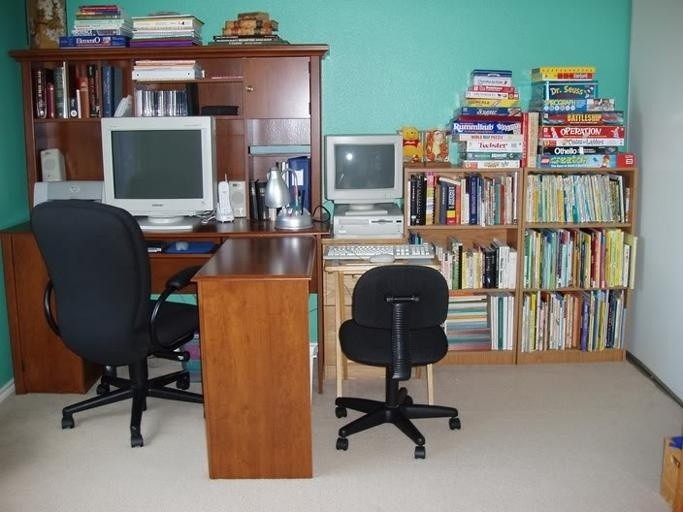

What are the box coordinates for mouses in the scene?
[370,254,395,263]
[176,240,189,251]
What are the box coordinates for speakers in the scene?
[228,181,247,218]
[40,148,67,182]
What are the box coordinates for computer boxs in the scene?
[332,204,405,240]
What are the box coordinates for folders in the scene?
[289,158,309,212]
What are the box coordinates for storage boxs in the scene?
[659,437,683,511]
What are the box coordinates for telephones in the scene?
[215,173,234,223]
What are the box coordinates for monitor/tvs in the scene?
[101,116,218,231]
[326,134,404,215]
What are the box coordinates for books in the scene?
[522,289,626,353]
[58,4,290,48]
[529,68,635,168]
[250,161,289,222]
[408,228,517,290]
[527,172,631,224]
[523,226,638,289]
[33,58,208,119]
[407,171,518,226]
[446,69,524,168]
[445,295,513,351]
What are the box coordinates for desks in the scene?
[323,238,438,406]
[189,237,324,480]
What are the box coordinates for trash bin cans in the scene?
[180,331,202,383]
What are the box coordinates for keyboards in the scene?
[323,243,435,262]
[145,240,166,252]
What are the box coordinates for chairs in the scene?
[332,264,461,461]
[30,200,204,448]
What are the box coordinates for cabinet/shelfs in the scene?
[3,43,333,396]
[403,163,523,367]
[517,168,636,365]
